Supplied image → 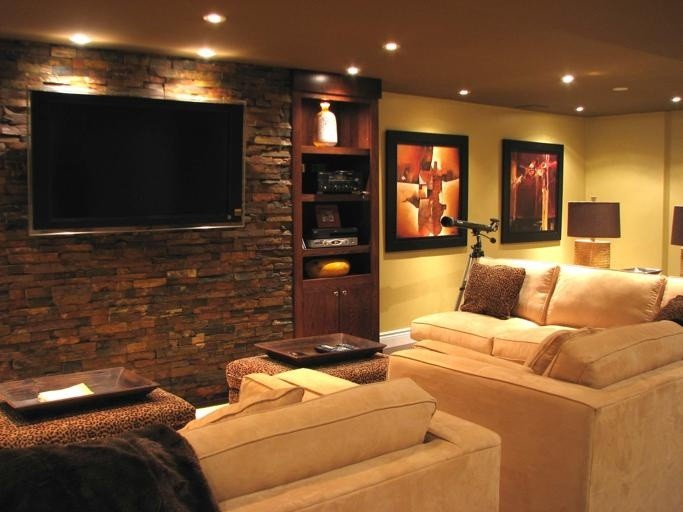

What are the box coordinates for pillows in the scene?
[460,261,526,319]
[652,294,683,326]
[526,327,606,372]
[175,388,304,437]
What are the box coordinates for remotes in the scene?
[316,344,352,352]
[337,344,360,350]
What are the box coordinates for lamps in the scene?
[671,205,683,277]
[566,200,622,268]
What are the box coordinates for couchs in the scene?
[1,365,501,512]
[410,253,683,362]
[388,347,681,511]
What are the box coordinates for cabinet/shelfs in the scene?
[291,91,380,338]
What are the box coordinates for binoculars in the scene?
[440,215,501,256]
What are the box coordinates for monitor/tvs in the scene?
[26,87,247,239]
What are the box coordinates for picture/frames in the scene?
[501,137,563,245]
[384,129,468,251]
